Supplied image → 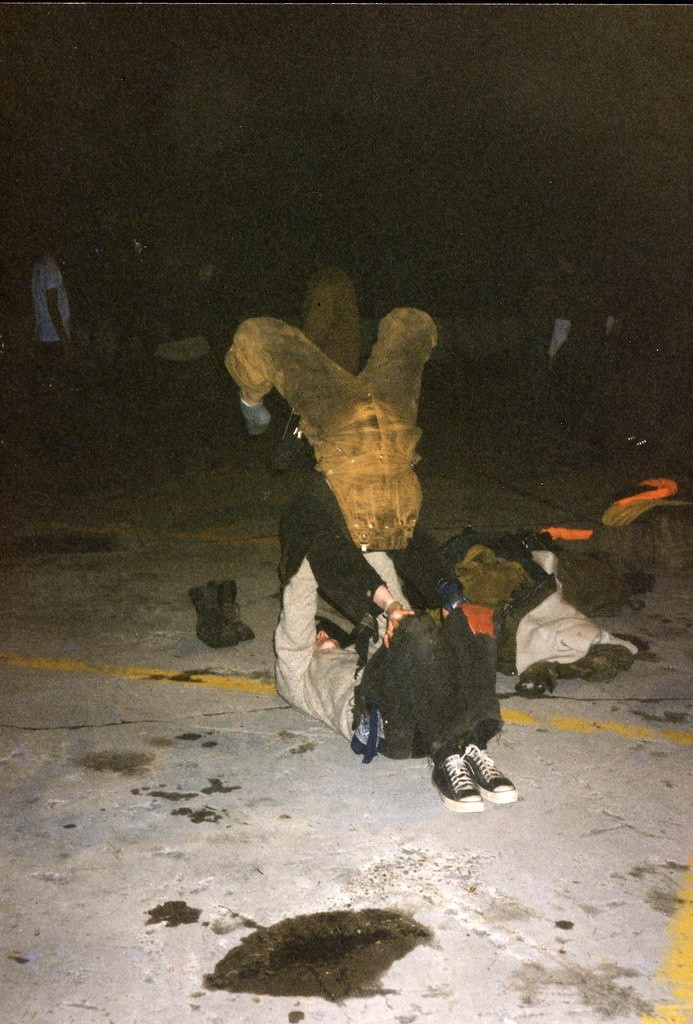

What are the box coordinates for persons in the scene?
[30,227,626,494]
[223,308,517,813]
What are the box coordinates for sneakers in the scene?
[431,753,486,813]
[462,744,518,804]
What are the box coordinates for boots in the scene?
[218,578,254,642]
[188,580,236,648]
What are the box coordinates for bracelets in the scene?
[383,599,400,617]
